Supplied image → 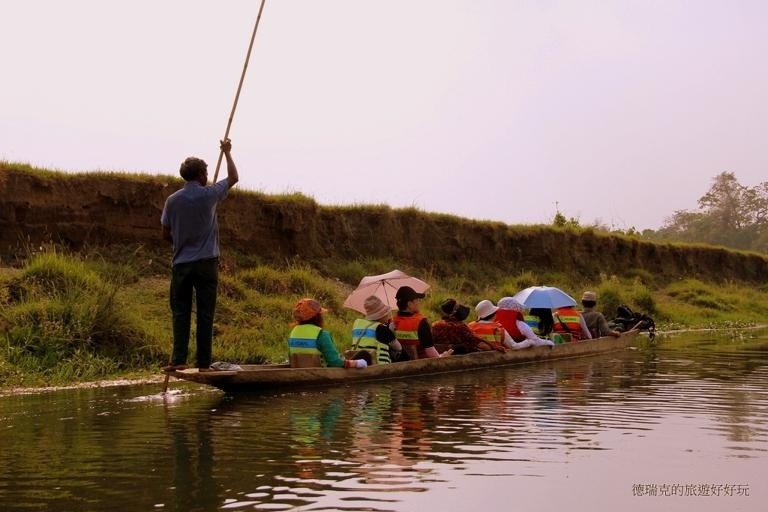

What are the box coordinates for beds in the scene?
[512,286,577,309]
[342,269,431,319]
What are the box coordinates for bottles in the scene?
[581,292,595,301]
[294,298,327,321]
[474,300,499,319]
[395,286,424,302]
[364,296,390,321]
[498,297,525,312]
[441,299,470,321]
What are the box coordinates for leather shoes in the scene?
[355,359,367,368]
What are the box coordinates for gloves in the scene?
[159,325,641,392]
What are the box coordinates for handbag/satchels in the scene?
[345,359,350,369]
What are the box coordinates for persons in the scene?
[287,297,368,369]
[159,138,238,373]
[552,306,593,340]
[579,291,626,339]
[524,308,566,345]
[467,300,534,350]
[430,298,491,354]
[171,413,217,511]
[352,296,402,365]
[388,286,454,358]
[495,297,556,349]
